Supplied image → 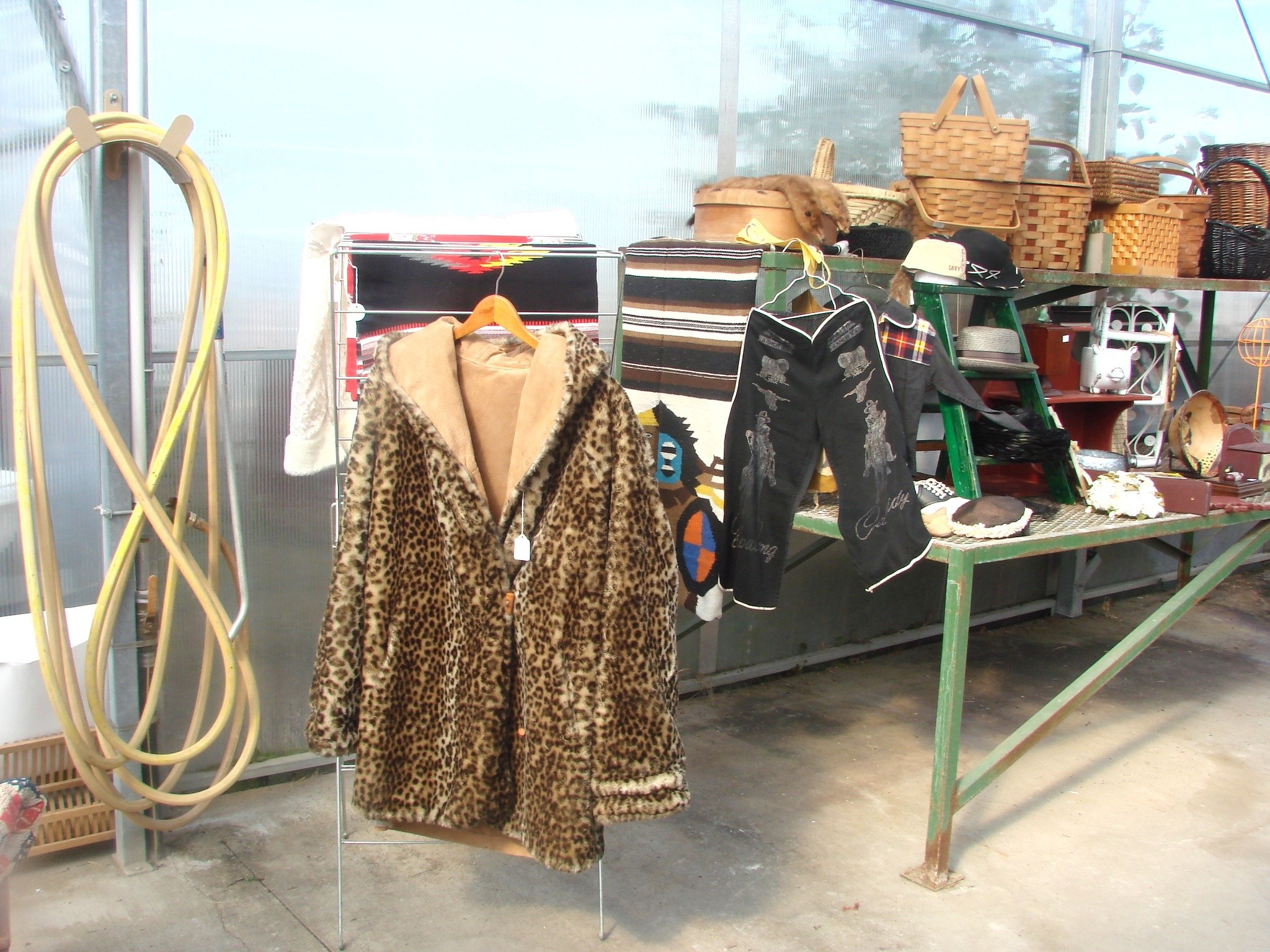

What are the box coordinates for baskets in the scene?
[1006,137,1093,271]
[810,137,909,234]
[891,178,1020,243]
[1088,198,1183,278]
[1199,143,1270,179]
[1196,162,1270,229]
[1127,156,1214,278]
[899,74,1030,184]
[1072,159,1160,204]
[1194,156,1270,279]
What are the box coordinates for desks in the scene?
[609,252,1270,893]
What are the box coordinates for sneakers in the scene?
[913,480,955,514]
[926,478,963,501]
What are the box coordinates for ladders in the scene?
[910,281,1078,506]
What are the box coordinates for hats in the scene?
[900,238,967,282]
[968,405,1070,464]
[927,228,1025,290]
[955,326,1040,374]
[949,494,1034,538]
